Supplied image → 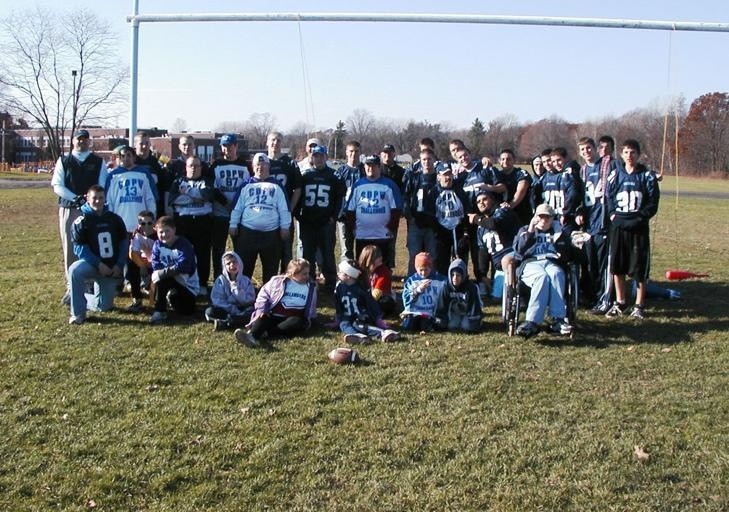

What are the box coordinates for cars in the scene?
[38,167,48,173]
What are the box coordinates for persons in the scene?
[61,184,129,325]
[107,131,662,350]
[51,130,107,305]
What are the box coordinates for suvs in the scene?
[26,165,39,171]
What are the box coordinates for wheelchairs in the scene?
[500,225,581,340]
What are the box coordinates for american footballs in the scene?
[328,349,359,363]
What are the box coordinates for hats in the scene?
[536,204,554,217]
[219,135,236,146]
[365,144,394,164]
[73,130,89,138]
[305,138,327,156]
[253,153,269,165]
[436,162,452,174]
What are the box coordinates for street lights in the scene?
[72,69,77,134]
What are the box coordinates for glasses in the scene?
[141,222,152,225]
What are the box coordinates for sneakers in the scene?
[126,303,143,313]
[149,311,168,321]
[214,318,256,348]
[590,297,645,320]
[551,319,575,335]
[516,322,534,337]
[381,329,402,342]
[343,333,370,345]
[68,313,86,324]
